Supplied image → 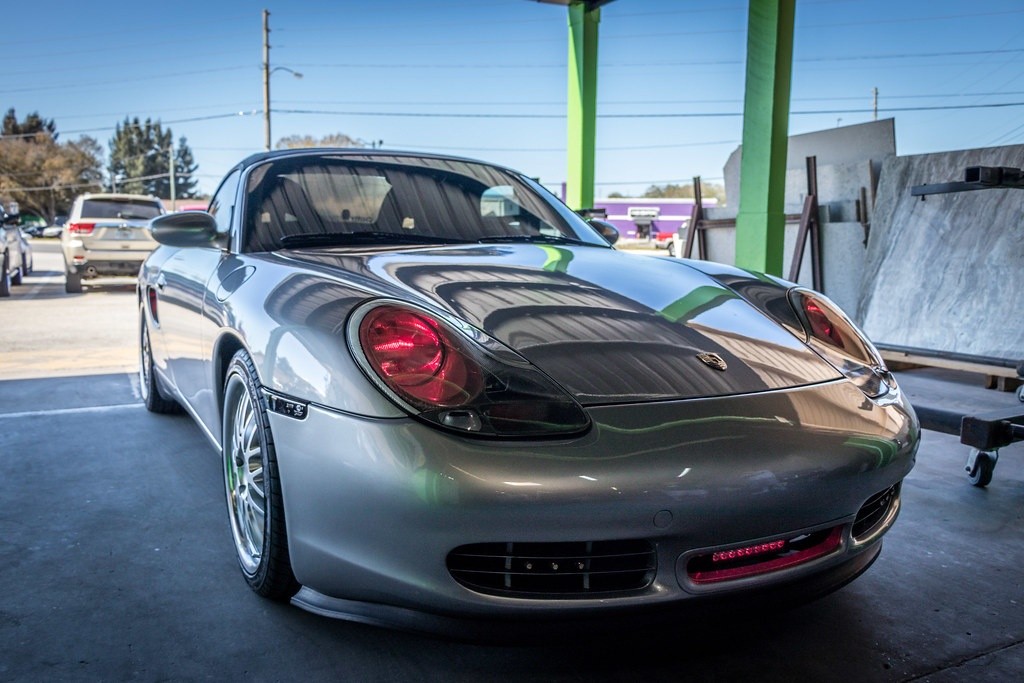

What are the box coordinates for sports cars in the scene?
[136,145,923,645]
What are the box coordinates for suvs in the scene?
[52,190,173,294]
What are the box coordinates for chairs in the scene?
[375,183,449,234]
[250,177,326,243]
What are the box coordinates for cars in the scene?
[15,209,71,240]
[0,204,33,299]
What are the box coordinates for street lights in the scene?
[262,65,305,153]
